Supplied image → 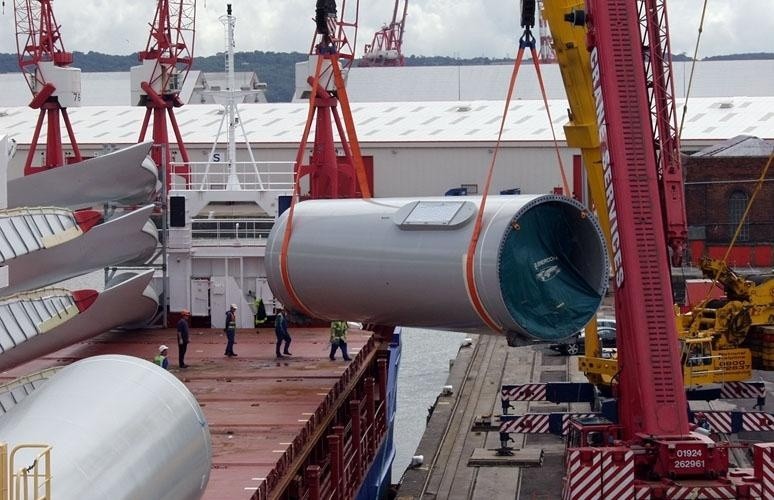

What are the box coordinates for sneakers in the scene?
[277,351,292,357]
[345,358,352,361]
[330,355,336,360]
[180,362,188,368]
[224,352,237,356]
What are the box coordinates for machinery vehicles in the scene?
[512,0,772,397]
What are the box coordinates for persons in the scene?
[176,309,190,368]
[274,307,292,358]
[329,320,351,360]
[152,344,169,370]
[223,303,237,357]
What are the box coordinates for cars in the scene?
[543,317,617,355]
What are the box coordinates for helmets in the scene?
[181,310,191,316]
[230,304,237,309]
[159,345,168,352]
